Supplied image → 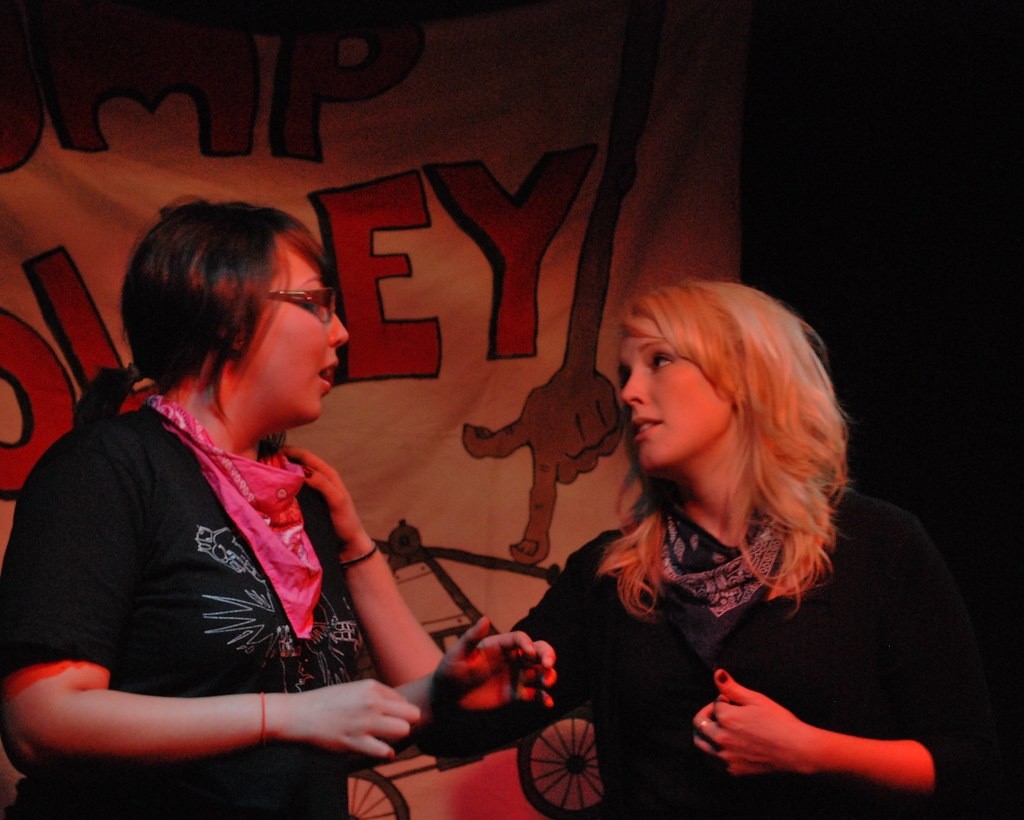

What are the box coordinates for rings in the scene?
[697,720,713,730]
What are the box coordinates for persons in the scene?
[0,201,558,820]
[281,281,1004,820]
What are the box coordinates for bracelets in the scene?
[338,541,378,568]
[261,692,266,745]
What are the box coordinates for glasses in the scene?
[267,286,337,322]
